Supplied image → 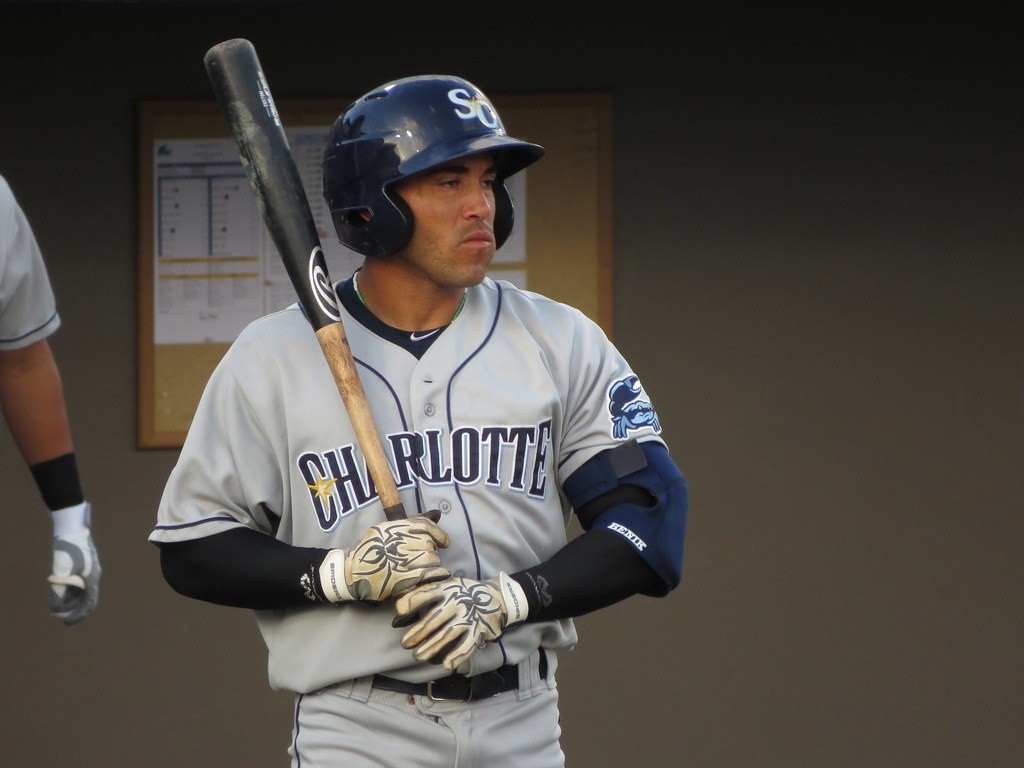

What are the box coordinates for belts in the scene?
[354,645,548,701]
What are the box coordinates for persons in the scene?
[148,73,688,765]
[0,174,100,625]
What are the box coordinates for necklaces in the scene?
[353,270,466,325]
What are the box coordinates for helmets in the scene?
[323,75,546,258]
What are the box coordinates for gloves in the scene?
[392,571,529,672]
[344,510,452,606]
[47,499,102,627]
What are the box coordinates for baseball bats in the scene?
[200,36,408,523]
